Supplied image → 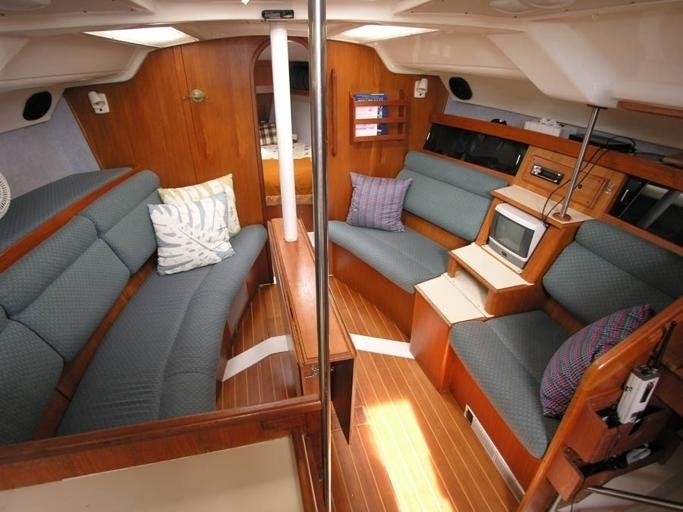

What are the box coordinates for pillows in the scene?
[541,305,649,417]
[347,169,413,235]
[148,169,240,277]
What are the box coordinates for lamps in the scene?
[415,80,427,99]
[86,91,110,115]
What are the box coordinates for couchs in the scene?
[0,166,268,446]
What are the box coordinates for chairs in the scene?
[446,217,683,494]
[328,147,507,344]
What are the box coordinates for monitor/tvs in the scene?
[489,202,546,270]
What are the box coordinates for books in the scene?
[352,94,388,138]
